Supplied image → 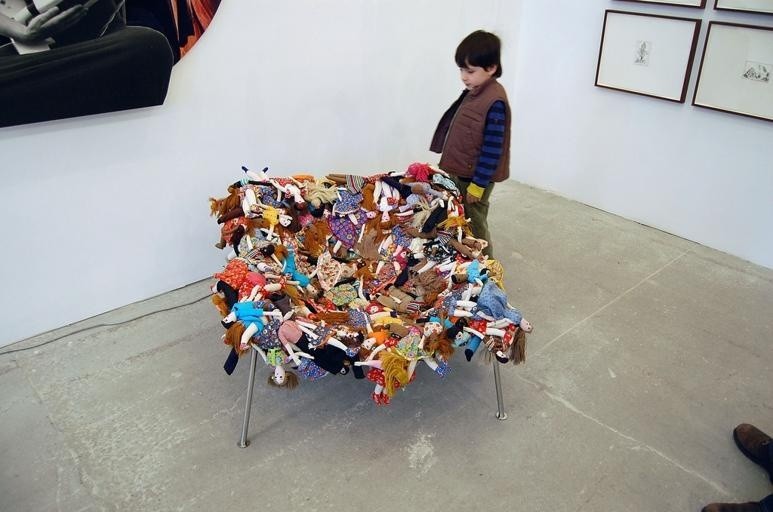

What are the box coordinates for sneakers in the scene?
[735,423,773,472]
[703,502,762,512]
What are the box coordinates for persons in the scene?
[702,424,772,509]
[429,30,510,260]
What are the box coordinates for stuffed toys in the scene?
[209,163,532,405]
[209,162,533,406]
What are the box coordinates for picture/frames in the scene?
[593,0,773,122]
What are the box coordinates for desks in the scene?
[212,168,526,449]
